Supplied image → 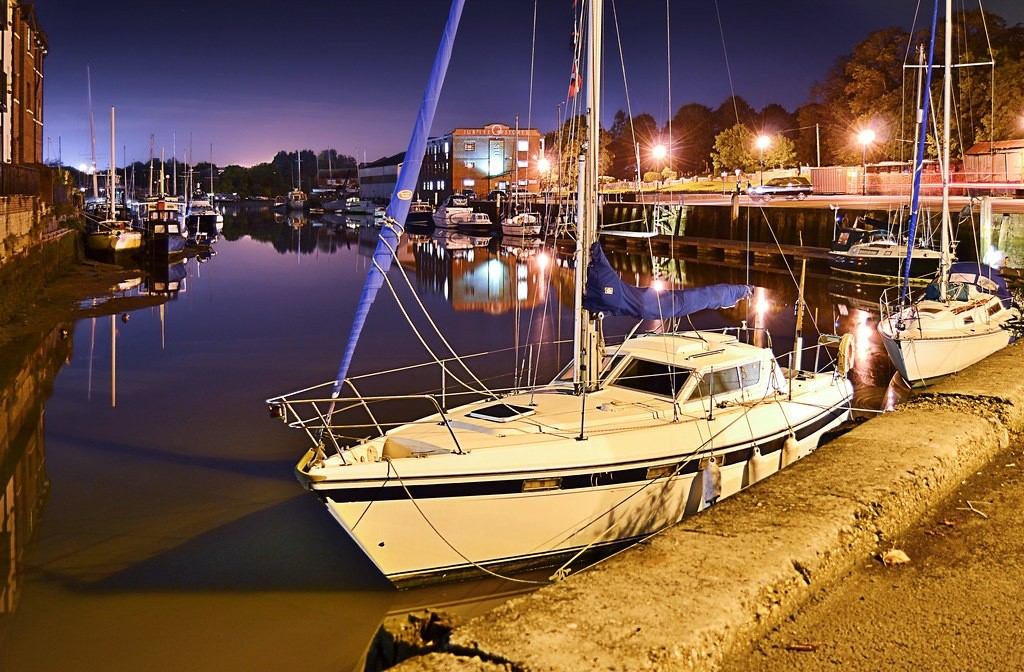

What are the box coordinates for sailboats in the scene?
[84,106,224,254]
[285,147,359,212]
[877,0,1021,389]
[262,0,856,588]
[88,244,216,407]
[499,112,544,236]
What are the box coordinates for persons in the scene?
[747,179,752,190]
[737,181,741,195]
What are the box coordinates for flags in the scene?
[567,0,582,101]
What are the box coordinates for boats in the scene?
[321,213,384,230]
[273,196,286,208]
[430,188,476,229]
[433,228,494,263]
[321,194,387,216]
[456,211,493,230]
[828,227,959,283]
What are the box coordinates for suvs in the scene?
[746,175,813,202]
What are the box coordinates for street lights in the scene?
[652,145,667,191]
[862,130,875,167]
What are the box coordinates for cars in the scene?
[462,189,538,200]
